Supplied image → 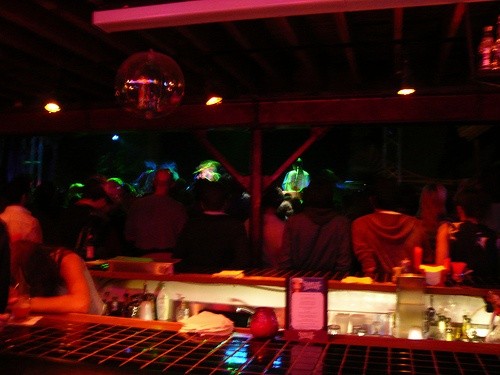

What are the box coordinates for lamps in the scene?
[395,43,419,96]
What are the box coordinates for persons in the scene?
[281,177,352,277]
[351,180,435,278]
[125,170,186,259]
[2,164,500,291]
[1,204,111,317]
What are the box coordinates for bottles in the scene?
[436,313,478,343]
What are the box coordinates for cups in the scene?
[9,282,31,321]
[140,301,152,321]
[329,313,366,335]
[397,273,424,340]
[425,269,441,285]
